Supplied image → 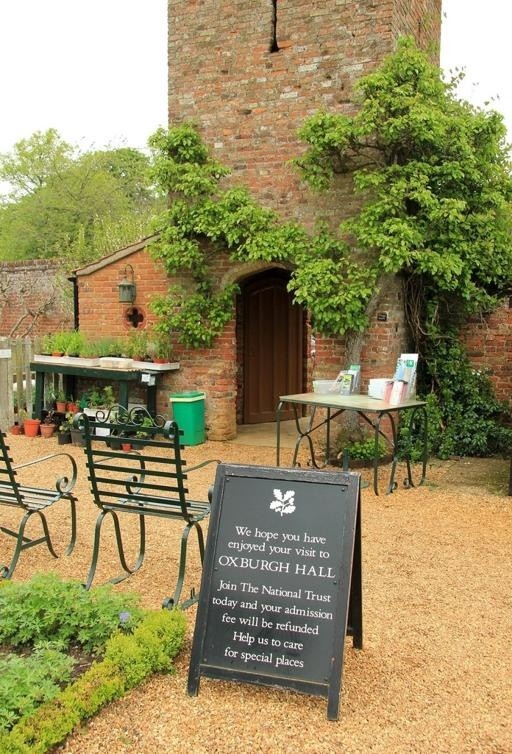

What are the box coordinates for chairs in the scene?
[72,406,223,611]
[0,428,77,584]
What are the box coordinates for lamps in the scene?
[118,264,137,305]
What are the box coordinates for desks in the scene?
[276,392,428,494]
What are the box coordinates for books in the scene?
[329,353,419,406]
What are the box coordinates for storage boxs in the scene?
[366,378,393,399]
[312,379,342,395]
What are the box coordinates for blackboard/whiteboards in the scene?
[191,464,362,696]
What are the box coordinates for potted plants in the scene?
[11,325,175,453]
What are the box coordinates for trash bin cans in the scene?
[169,392,206,446]
